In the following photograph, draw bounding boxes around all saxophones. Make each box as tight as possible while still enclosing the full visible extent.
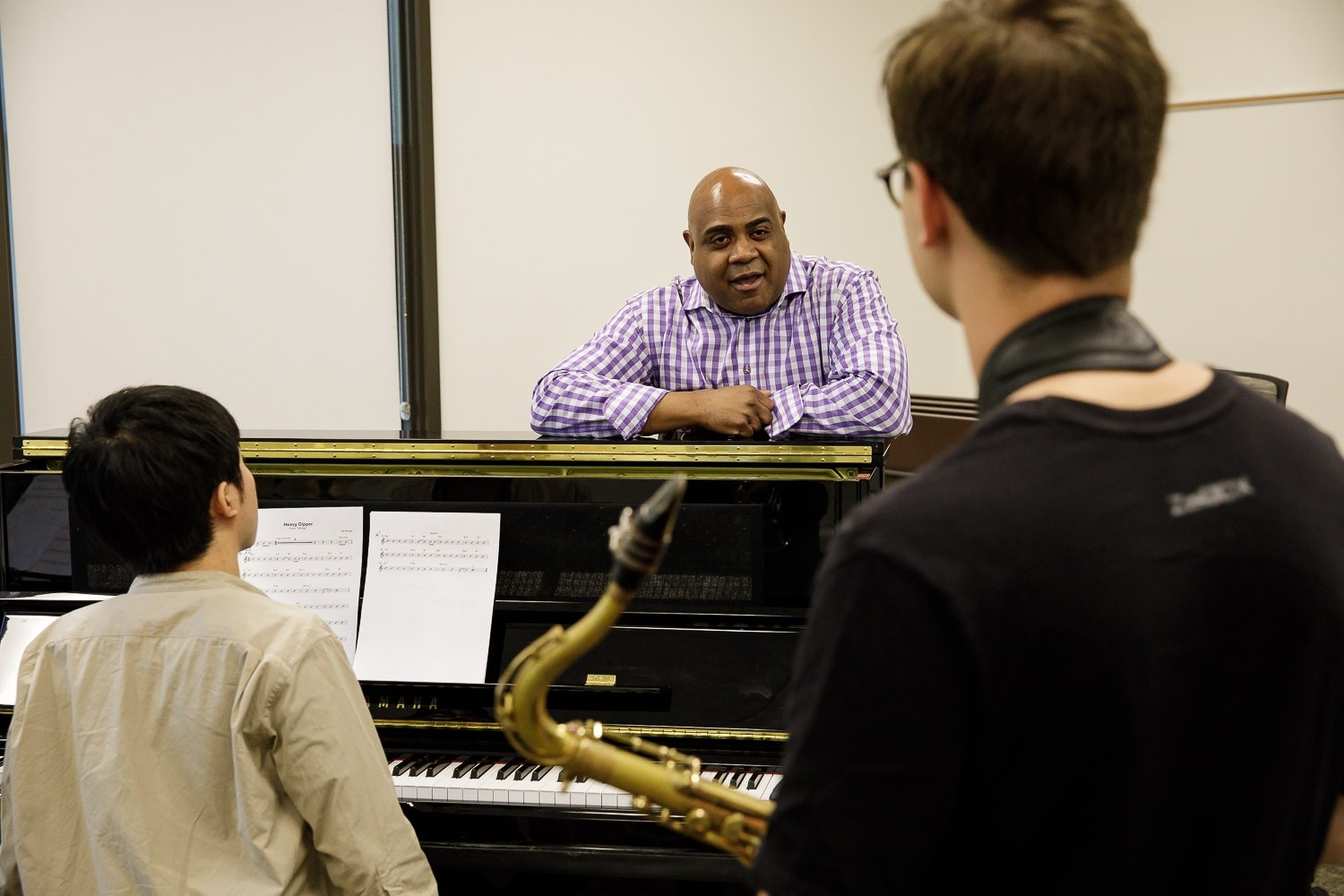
[493,465,770,869]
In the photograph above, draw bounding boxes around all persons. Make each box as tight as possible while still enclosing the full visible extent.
[0,384,438,896]
[750,0,1344,896]
[530,166,913,438]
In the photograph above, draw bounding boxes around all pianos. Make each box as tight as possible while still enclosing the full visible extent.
[54,428,892,894]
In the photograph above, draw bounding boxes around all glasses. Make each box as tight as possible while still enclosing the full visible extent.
[878,155,938,210]
